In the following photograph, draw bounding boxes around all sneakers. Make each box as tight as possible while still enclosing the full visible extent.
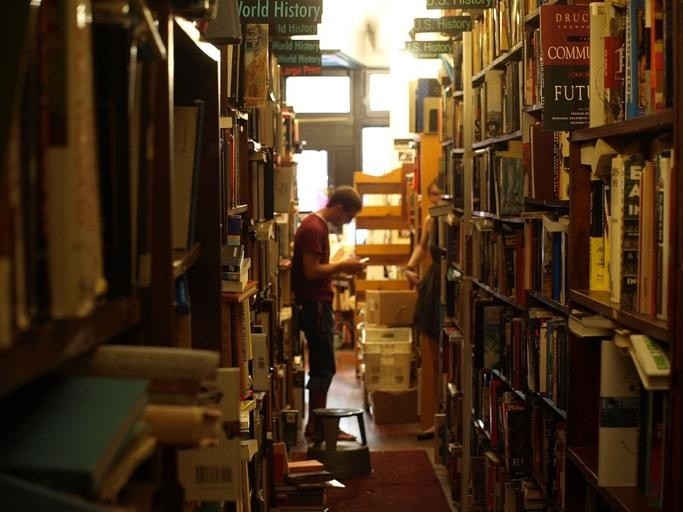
[416,429,436,441]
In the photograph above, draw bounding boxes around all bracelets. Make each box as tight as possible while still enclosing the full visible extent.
[405,264,415,271]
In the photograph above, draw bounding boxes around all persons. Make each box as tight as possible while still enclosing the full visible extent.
[402,172,439,441]
[291,184,368,443]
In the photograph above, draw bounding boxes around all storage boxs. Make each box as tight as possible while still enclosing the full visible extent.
[361,324,415,392]
[360,287,417,324]
[367,390,420,426]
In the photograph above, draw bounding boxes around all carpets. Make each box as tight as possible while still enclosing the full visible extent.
[289,449,451,511]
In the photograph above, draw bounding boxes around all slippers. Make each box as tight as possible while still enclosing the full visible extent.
[305,426,358,442]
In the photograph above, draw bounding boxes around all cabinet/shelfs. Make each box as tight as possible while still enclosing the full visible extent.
[354,132,413,429]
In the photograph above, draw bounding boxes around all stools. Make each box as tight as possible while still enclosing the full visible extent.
[312,405,374,479]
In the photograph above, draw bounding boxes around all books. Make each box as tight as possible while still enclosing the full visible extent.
[0,0,349,511]
[437,0,676,511]
